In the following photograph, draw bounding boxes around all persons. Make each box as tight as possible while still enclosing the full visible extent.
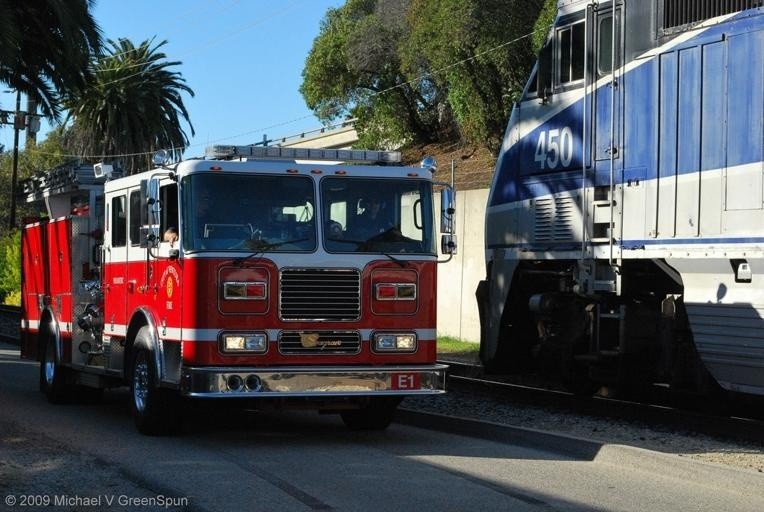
[344,195,393,234]
[162,187,226,249]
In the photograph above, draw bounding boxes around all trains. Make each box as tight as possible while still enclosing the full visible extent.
[474,0,764,406]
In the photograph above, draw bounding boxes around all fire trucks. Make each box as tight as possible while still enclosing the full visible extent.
[17,138,463,438]
